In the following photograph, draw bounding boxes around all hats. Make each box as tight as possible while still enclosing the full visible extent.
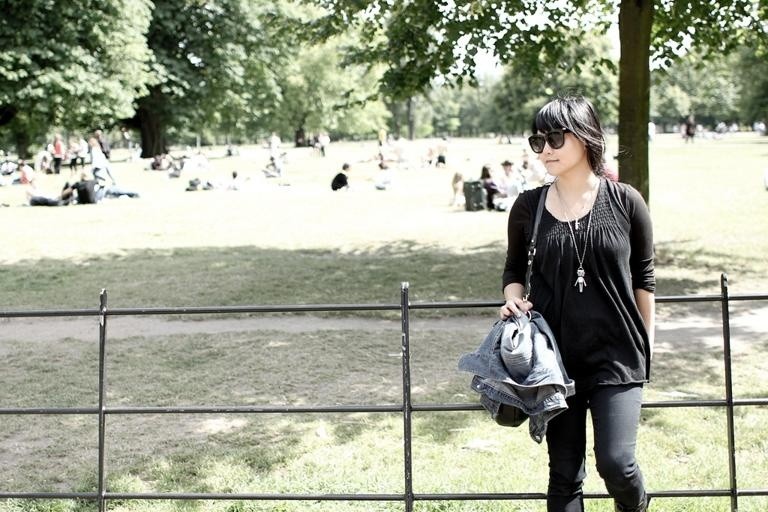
[502,161,511,165]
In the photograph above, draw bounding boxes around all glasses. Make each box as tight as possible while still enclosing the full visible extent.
[528,128,571,152]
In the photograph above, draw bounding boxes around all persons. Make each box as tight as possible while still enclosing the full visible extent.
[646,112,768,144]
[1,127,542,213]
[498,91,658,512]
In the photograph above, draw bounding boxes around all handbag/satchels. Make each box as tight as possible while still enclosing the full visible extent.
[480,394,529,428]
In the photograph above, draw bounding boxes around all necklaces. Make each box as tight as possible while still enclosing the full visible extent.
[554,175,597,295]
